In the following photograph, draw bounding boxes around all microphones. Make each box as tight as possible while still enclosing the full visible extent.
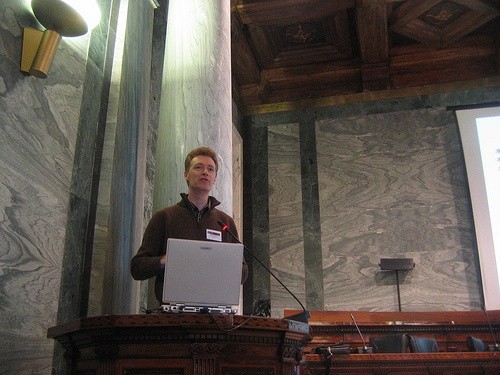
[350,314,373,353]
[484,307,500,351]
[216,219,311,323]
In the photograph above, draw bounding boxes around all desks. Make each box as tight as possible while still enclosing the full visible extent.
[47,313,309,375]
[304,351,500,375]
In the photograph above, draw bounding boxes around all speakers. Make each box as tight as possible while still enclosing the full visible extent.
[380,258,414,270]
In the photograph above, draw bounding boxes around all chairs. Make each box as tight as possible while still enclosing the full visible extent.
[466,335,486,352]
[371,334,409,353]
[410,336,439,352]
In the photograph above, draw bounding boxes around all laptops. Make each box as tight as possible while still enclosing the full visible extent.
[145,238,243,314]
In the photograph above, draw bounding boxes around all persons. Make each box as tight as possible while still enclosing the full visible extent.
[131,147,248,308]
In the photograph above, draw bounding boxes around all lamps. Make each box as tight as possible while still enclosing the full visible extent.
[20,0,102,79]
